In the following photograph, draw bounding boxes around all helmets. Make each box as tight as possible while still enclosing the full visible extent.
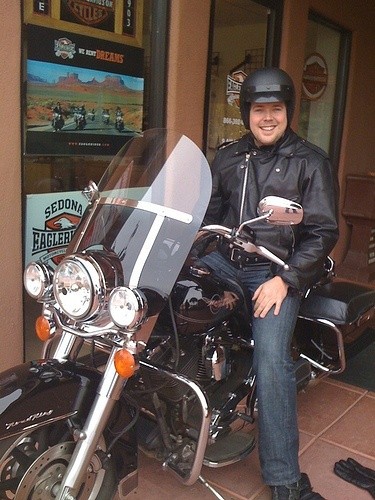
[240,67,295,129]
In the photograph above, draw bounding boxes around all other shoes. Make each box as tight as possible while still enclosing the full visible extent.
[270,473,326,500]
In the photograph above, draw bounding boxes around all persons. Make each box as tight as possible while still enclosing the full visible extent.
[161,66,341,500]
[52,102,123,129]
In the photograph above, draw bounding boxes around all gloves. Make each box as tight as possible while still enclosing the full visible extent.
[334,458,375,499]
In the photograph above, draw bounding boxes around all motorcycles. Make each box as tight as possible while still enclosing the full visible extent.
[73,112,110,130]
[0,128,375,500]
[50,107,66,131]
[114,114,125,132]
